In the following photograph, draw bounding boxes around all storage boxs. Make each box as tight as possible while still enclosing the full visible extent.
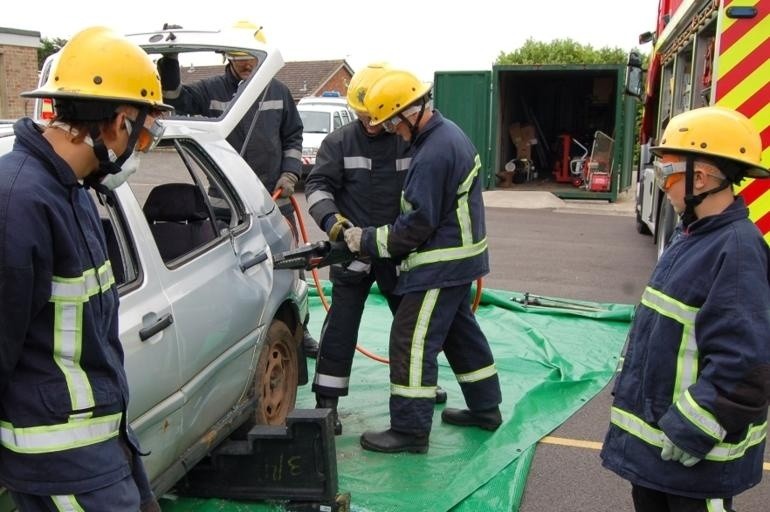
[510,122,537,160]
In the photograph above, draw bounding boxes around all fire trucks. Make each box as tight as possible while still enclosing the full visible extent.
[621,0,769,269]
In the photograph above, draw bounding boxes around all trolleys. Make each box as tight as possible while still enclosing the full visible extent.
[566,135,588,188]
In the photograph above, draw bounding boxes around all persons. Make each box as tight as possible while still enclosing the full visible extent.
[342,69,503,458]
[158,21,320,360]
[304,64,451,438]
[599,107,770,512]
[0,27,177,512]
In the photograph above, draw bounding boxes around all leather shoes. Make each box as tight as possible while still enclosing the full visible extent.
[436,389,447,407]
[441,408,500,432]
[360,430,429,455]
[303,330,320,359]
[318,407,342,436]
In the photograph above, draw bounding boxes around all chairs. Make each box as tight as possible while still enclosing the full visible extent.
[106,183,229,283]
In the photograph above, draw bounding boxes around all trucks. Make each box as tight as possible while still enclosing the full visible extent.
[293,88,360,182]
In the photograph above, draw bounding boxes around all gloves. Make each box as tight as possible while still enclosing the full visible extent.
[276,172,298,198]
[323,214,353,242]
[344,227,362,253]
[156,56,180,91]
[660,432,701,467]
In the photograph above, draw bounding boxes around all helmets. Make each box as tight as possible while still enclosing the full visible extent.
[224,22,267,59]
[346,61,391,115]
[21,25,176,115]
[648,106,769,178]
[362,69,435,126]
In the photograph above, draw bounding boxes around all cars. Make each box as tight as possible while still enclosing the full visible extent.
[1,22,321,511]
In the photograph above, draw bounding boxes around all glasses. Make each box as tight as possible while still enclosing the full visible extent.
[224,55,260,71]
[653,153,726,191]
[382,101,433,134]
[112,104,165,153]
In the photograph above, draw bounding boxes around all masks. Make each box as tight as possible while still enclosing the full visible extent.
[100,151,140,190]
